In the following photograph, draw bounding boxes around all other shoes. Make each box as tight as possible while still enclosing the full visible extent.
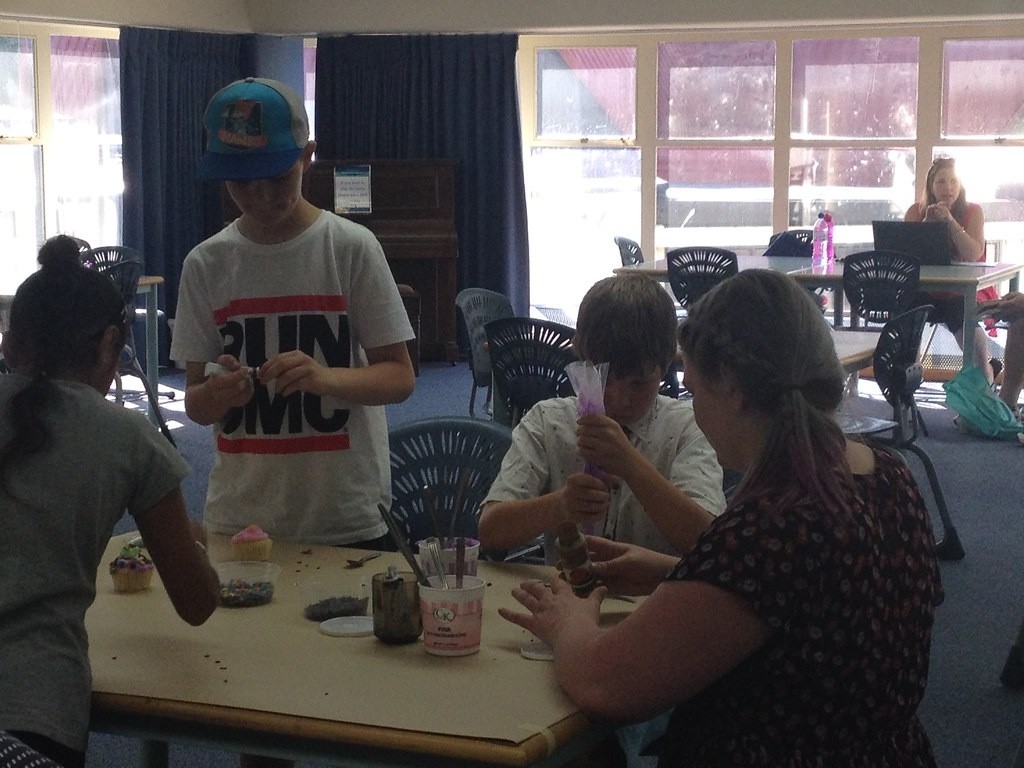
[951,404,1020,434]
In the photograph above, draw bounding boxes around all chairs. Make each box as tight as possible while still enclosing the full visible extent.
[53,228,1003,565]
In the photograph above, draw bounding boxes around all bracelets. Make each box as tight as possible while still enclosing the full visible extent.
[952,227,965,242]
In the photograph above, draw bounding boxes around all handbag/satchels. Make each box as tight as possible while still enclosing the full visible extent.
[942,365,1024,441]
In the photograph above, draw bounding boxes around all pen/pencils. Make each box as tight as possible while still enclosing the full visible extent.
[429,544,450,589]
[377,503,433,588]
[448,469,471,547]
[456,538,466,589]
[422,490,446,550]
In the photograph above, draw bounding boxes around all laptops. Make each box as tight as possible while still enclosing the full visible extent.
[872,220,952,265]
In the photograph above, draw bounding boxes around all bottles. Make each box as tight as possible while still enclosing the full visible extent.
[812,213,828,262]
[555,522,597,594]
[824,214,834,259]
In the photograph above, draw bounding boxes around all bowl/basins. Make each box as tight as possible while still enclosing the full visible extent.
[215,560,282,607]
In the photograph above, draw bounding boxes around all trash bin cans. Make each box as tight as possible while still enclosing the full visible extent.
[655,177,672,226]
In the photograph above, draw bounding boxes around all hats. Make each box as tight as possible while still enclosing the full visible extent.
[200,76,309,181]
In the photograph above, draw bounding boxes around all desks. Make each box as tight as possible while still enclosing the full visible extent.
[483,315,688,399]
[135,274,164,429]
[612,255,845,328]
[789,260,1024,433]
[85,525,665,768]
[830,331,883,393]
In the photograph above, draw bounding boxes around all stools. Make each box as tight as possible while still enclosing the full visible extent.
[396,283,423,378]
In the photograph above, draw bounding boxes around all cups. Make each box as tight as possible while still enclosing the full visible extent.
[419,536,481,577]
[418,575,486,657]
[372,571,423,645]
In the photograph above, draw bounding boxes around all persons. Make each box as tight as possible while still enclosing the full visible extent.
[477,272,726,557]
[0,235,222,767]
[873,157,1024,428]
[497,268,938,768]
[170,76,416,552]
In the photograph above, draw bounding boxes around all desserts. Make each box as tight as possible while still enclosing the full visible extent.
[109,546,154,592]
[230,524,273,561]
